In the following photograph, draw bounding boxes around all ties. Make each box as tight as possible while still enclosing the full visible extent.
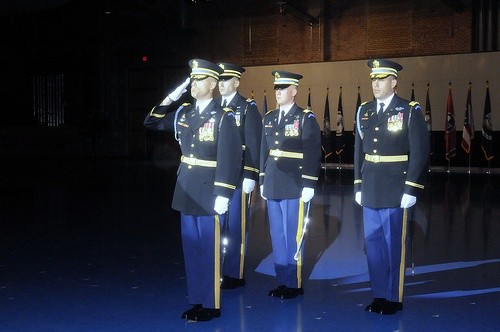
[195,107,199,114]
[280,110,285,119]
[377,103,385,124]
[222,100,227,107]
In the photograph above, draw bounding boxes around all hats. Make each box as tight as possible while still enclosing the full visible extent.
[215,63,245,81]
[367,58,403,79]
[188,59,224,81]
[272,70,304,89]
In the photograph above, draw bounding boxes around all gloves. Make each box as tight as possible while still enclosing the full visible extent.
[259,185,267,201]
[302,186,314,203]
[168,77,190,102]
[400,193,417,208]
[355,191,363,206]
[214,195,229,215]
[243,178,256,194]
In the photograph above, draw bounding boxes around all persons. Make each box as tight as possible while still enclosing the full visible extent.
[144,58,242,322]
[353,57,430,315]
[215,62,263,289]
[259,71,321,300]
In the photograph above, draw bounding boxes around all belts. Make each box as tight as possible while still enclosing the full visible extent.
[269,149,303,159]
[364,154,408,163]
[180,155,217,167]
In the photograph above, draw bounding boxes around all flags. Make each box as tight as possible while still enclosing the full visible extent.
[461,86,475,153]
[250,88,432,154]
[445,87,457,160]
[481,84,493,165]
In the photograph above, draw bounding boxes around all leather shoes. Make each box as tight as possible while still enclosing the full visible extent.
[181,304,201,319]
[191,307,219,322]
[279,287,305,300]
[268,285,286,296]
[364,297,385,312]
[219,275,244,290]
[380,300,403,315]
